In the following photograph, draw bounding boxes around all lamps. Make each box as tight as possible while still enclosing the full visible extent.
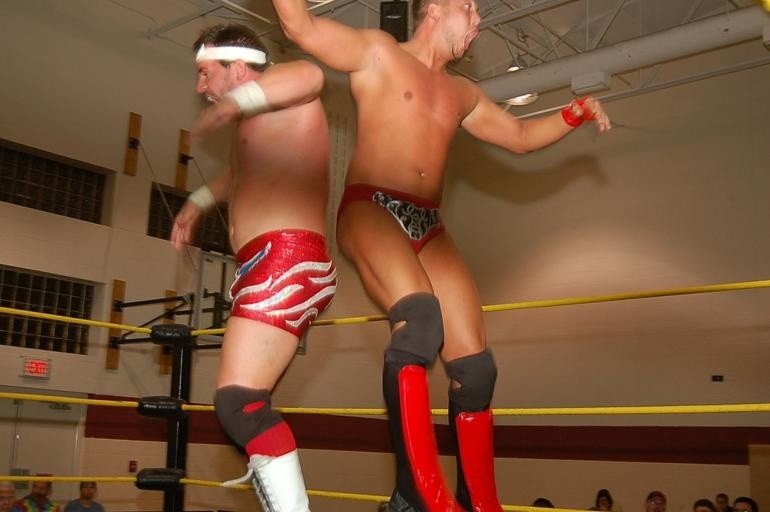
[499,59,538,106]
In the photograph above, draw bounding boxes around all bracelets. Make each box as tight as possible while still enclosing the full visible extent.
[222,79,269,118]
[188,184,217,214]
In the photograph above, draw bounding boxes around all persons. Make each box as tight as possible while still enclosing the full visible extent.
[63,480,105,512]
[693,492,759,511]
[11,474,61,512]
[166,22,340,512]
[588,488,612,511]
[271,1,612,512]
[645,491,667,512]
